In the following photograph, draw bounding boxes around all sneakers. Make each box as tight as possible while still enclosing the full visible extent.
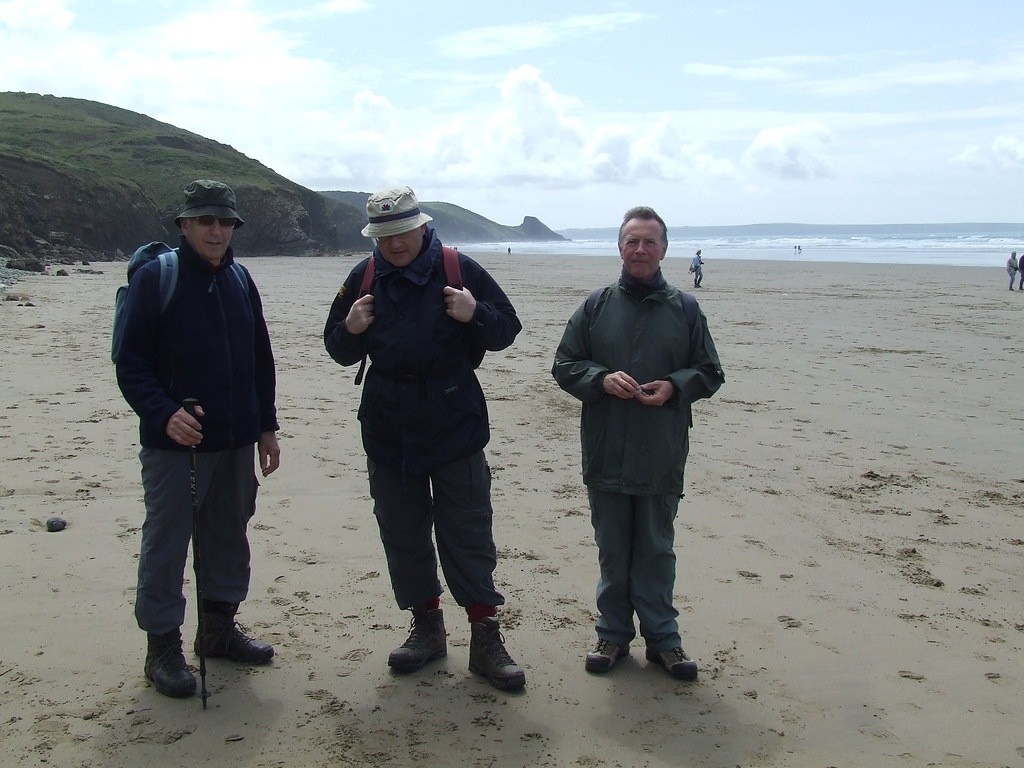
[585,638,629,673]
[646,646,698,681]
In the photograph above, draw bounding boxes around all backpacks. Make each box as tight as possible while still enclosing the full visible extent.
[111,242,249,364]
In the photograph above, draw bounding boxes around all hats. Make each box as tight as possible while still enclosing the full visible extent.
[696,249,701,255]
[174,180,245,230]
[361,186,433,238]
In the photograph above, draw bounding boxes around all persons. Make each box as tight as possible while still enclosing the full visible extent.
[552,206,725,682]
[692,248,704,288]
[1007,251,1024,291]
[111,179,280,699]
[508,247,511,255]
[322,186,526,690]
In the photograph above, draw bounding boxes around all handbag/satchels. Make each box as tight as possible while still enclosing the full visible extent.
[1015,267,1018,271]
[689,267,697,272]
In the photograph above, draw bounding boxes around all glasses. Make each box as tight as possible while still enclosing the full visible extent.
[190,215,237,226]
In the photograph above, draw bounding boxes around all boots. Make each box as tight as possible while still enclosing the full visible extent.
[194,598,274,665]
[694,278,702,288]
[144,629,197,698]
[468,616,526,693]
[388,609,447,673]
